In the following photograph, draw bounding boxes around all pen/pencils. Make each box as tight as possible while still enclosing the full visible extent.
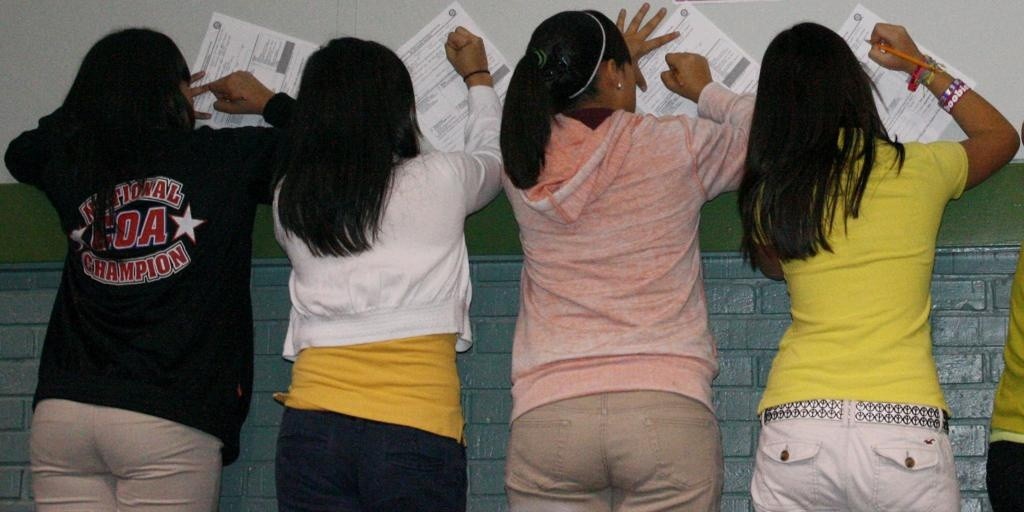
[670,65,685,86]
[223,96,247,101]
[455,41,472,51]
[864,39,935,71]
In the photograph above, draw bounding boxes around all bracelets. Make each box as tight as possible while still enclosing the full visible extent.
[464,70,491,82]
[937,80,970,114]
[908,50,933,91]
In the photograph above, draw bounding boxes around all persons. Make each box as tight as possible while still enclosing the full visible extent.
[736,21,1020,512]
[497,3,755,512]
[985,240,1024,512]
[271,27,504,511]
[4,28,296,512]
